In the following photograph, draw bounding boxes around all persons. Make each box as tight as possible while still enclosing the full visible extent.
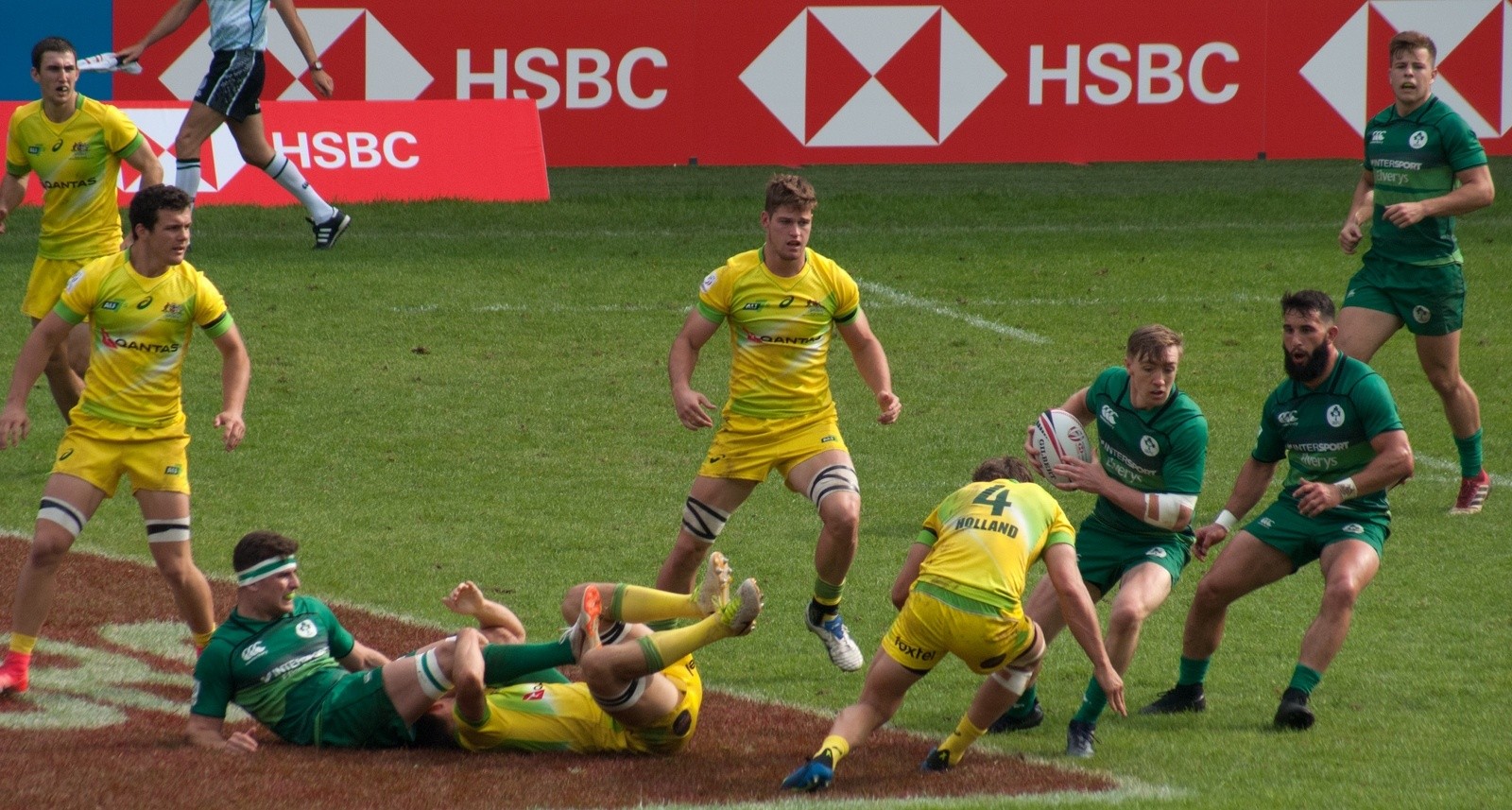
[1137,289,1415,732]
[1333,30,1496,514]
[109,0,352,255]
[779,456,1128,793]
[646,174,902,672]
[0,184,251,695]
[186,530,765,759]
[0,36,164,426]
[985,324,1210,759]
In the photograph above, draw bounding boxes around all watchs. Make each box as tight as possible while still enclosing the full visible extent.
[309,60,323,71]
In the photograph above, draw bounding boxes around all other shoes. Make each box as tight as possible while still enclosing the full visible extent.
[0,660,27,696]
[197,647,203,657]
[558,584,602,663]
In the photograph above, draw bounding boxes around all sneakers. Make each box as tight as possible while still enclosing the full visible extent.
[781,749,834,793]
[1449,470,1491,515]
[1065,720,1103,759]
[921,745,951,773]
[803,598,864,673]
[709,578,763,637]
[1137,682,1206,715]
[988,697,1044,734]
[1274,687,1315,731]
[304,207,349,250]
[686,551,732,617]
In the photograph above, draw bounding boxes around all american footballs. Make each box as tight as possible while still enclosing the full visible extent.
[1031,410,1093,490]
